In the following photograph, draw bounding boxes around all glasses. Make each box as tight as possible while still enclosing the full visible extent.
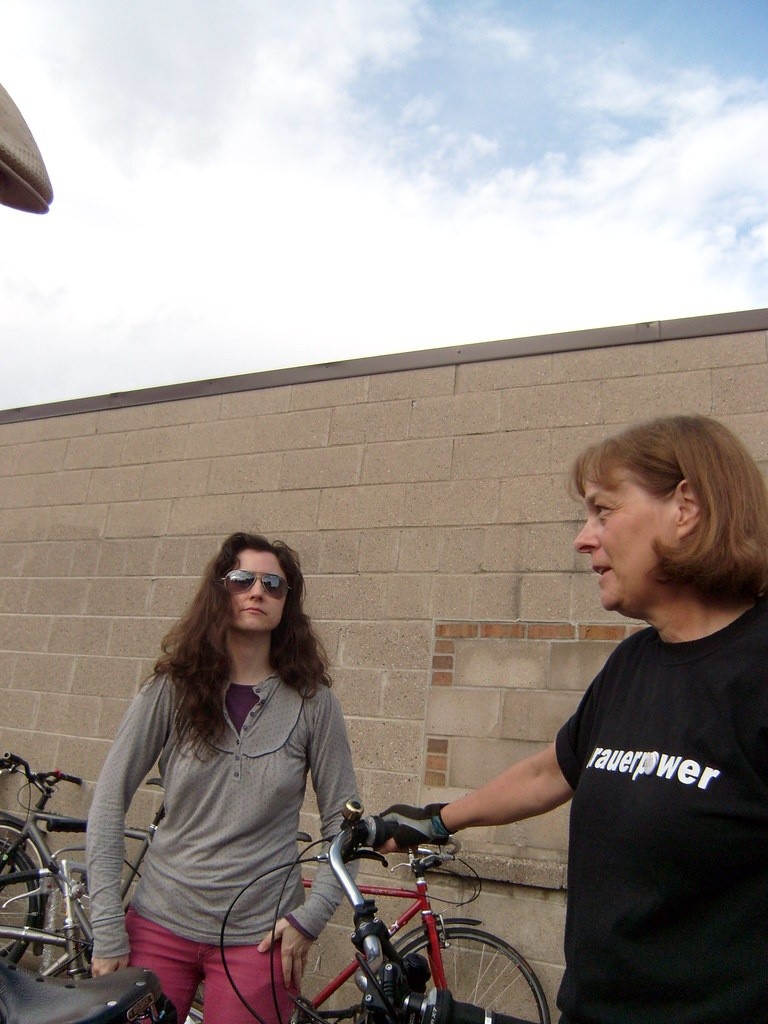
[220,569,292,600]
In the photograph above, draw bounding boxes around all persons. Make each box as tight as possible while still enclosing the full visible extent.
[377,416,768,1024]
[86,530,363,1024]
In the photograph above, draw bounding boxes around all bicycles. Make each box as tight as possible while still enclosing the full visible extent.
[0,748,553,1024]
